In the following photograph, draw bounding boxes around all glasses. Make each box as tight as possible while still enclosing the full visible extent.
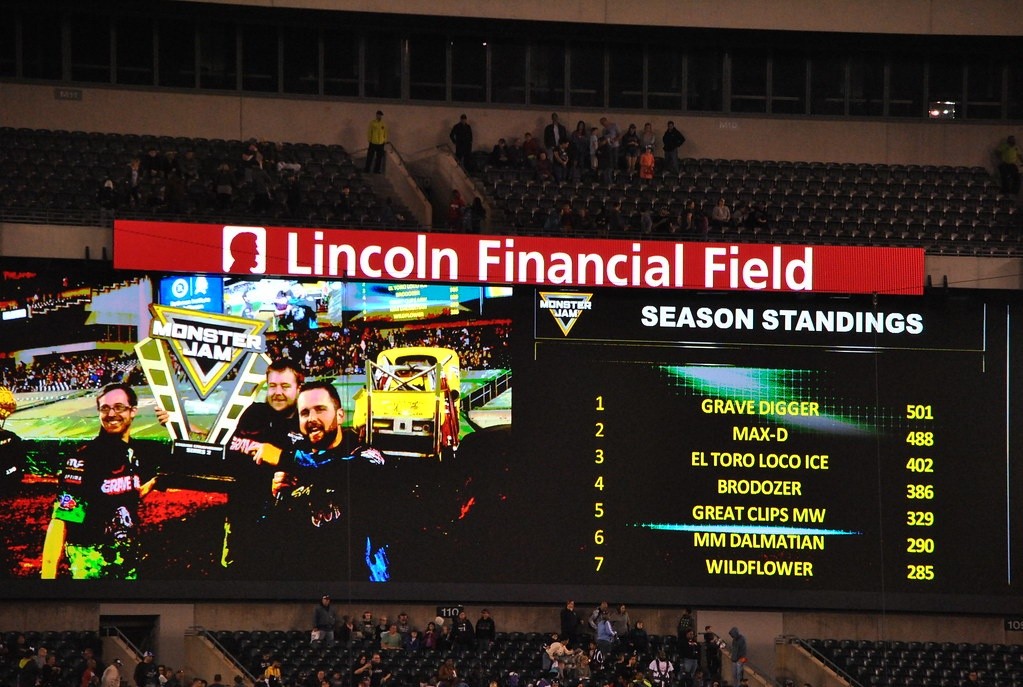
[98,403,132,413]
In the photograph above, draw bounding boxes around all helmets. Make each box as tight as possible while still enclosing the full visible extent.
[112,659,123,666]
[63,654,88,672]
[143,650,155,657]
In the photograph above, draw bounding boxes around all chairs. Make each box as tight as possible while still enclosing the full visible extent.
[0,123,421,232]
[803,639,1023,687]
[464,150,1023,257]
[0,630,105,687]
[202,628,682,687]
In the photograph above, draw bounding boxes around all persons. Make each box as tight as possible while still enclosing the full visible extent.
[448,190,486,232]
[711,198,730,222]
[545,200,710,240]
[662,121,685,160]
[509,139,523,169]
[544,113,656,184]
[535,152,554,183]
[450,114,473,160]
[0,593,747,687]
[0,268,509,560]
[997,135,1023,197]
[522,133,541,157]
[341,186,356,208]
[125,140,275,207]
[364,111,387,175]
[494,138,508,168]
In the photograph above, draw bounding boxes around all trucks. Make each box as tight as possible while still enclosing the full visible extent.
[352,347,463,454]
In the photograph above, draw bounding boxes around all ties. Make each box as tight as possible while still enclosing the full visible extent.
[128,447,134,463]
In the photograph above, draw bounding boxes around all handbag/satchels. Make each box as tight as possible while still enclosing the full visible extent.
[309,628,320,643]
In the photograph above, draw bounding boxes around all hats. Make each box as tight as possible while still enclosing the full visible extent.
[399,610,408,617]
